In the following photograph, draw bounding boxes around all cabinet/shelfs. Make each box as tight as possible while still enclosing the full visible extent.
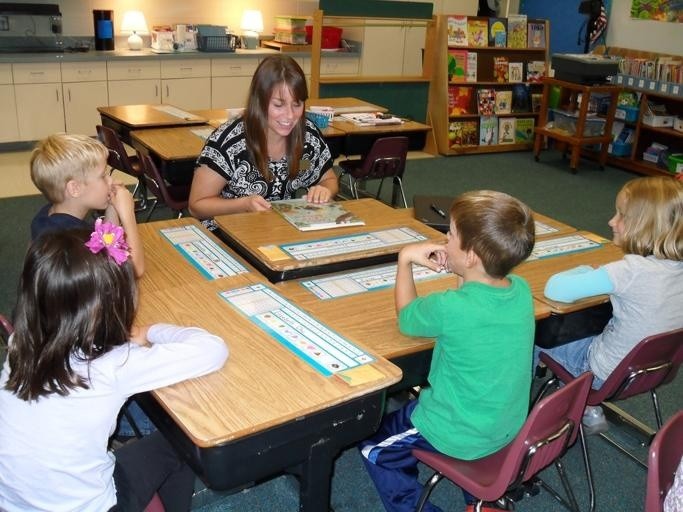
[104,60,211,113]
[423,15,552,157]
[0,63,18,149]
[260,57,358,95]
[324,13,430,79]
[10,60,107,143]
[207,58,259,111]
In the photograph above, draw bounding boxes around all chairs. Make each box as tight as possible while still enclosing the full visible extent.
[0,316,142,445]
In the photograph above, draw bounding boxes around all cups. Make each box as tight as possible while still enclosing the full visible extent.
[93,10,115,51]
[495,31,506,48]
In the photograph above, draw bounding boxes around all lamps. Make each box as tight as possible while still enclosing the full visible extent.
[236,9,266,49]
[118,10,148,50]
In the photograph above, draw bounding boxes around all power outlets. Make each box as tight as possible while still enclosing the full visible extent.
[48,16,64,35]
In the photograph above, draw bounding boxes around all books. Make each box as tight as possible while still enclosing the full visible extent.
[447,13,547,147]
[611,54,682,116]
[267,199,365,232]
[341,112,403,128]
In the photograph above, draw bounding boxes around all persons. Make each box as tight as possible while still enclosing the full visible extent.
[28,131,146,279]
[186,53,339,234]
[357,188,536,512]
[532,174,682,436]
[0,231,229,512]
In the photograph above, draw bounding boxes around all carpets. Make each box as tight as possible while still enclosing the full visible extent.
[0,150,683,510]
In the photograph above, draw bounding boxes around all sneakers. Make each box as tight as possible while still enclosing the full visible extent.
[576,401,613,441]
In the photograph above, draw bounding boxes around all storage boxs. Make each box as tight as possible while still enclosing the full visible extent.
[304,23,344,49]
[272,26,306,44]
[550,52,619,85]
[270,16,307,28]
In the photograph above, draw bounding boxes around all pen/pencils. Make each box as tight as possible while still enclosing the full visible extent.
[353,117,362,121]
[431,204,447,218]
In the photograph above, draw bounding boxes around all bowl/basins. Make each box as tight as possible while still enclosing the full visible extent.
[305,25,342,50]
